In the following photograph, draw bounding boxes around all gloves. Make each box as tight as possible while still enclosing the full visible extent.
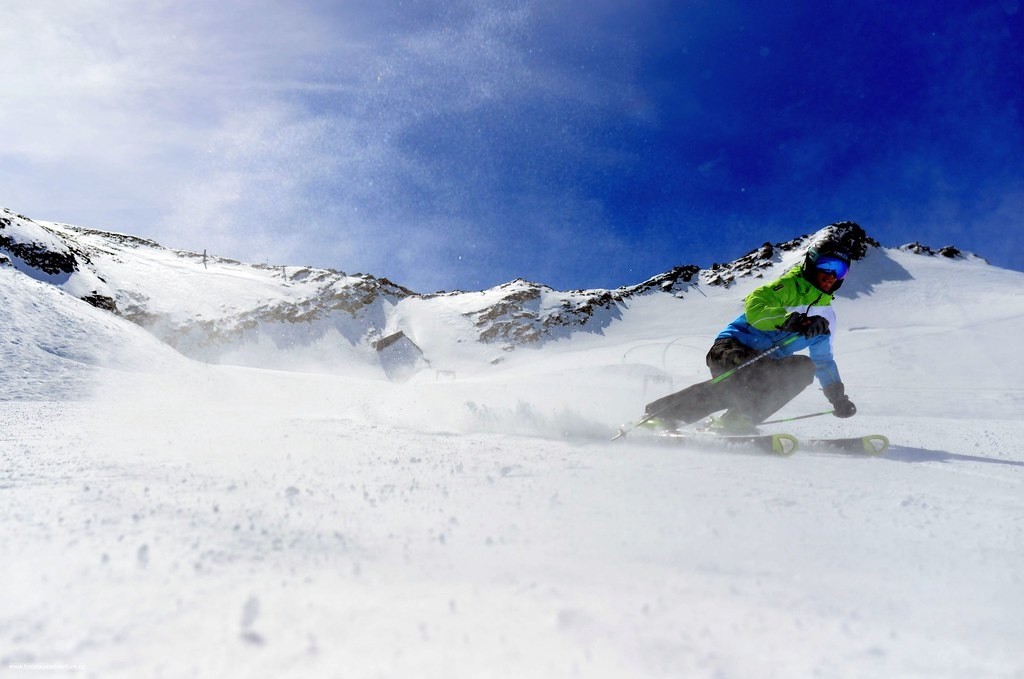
[776,311,830,341]
[822,383,857,418]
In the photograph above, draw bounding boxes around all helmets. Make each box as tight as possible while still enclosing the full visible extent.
[805,239,851,295]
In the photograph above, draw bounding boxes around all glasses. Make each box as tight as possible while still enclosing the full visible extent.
[814,253,850,281]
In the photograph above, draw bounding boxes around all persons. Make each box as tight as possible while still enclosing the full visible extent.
[639,240,857,436]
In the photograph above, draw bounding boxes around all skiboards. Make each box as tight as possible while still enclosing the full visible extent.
[617,434,887,455]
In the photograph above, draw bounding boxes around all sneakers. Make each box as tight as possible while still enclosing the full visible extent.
[715,413,760,435]
[635,413,676,433]
